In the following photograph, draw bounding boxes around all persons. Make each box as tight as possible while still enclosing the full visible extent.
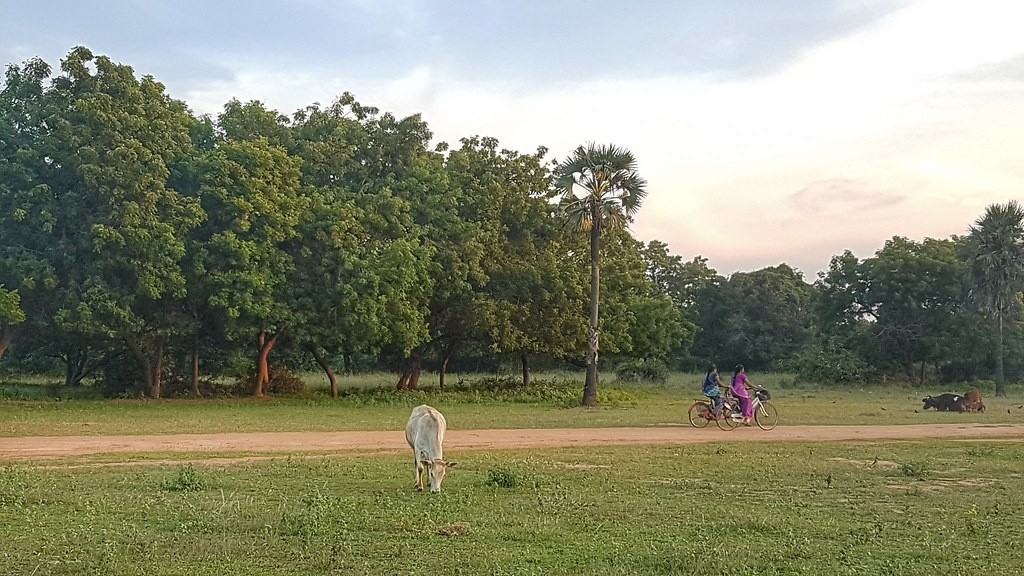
[702,364,732,419]
[731,364,764,427]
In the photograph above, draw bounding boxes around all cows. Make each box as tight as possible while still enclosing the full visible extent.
[405,404,459,494]
[922,390,986,414]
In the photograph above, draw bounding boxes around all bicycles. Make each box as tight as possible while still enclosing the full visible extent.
[714,386,779,430]
[687,387,746,428]
